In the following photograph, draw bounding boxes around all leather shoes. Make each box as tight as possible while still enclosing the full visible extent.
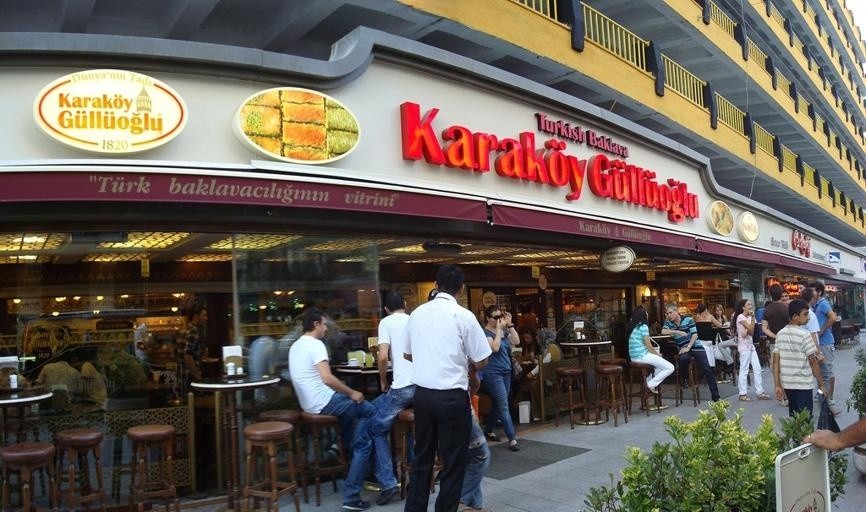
[509,440,520,451]
[484,431,499,442]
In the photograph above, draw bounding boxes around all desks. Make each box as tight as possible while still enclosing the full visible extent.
[1,386,56,440]
[559,341,613,427]
[333,360,394,398]
[187,372,284,511]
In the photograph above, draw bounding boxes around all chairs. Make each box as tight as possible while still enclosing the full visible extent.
[0,341,618,408]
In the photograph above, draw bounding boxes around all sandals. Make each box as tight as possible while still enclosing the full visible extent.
[739,393,771,401]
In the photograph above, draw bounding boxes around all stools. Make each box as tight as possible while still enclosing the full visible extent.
[555,364,591,431]
[49,426,105,510]
[392,405,444,499]
[629,359,661,417]
[242,419,300,509]
[603,358,629,412]
[259,409,309,503]
[598,364,629,428]
[125,422,181,512]
[1,440,55,512]
[674,352,701,409]
[729,345,752,387]
[299,409,348,508]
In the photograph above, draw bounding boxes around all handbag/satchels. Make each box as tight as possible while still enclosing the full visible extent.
[511,355,523,375]
[518,401,531,424]
[713,332,731,361]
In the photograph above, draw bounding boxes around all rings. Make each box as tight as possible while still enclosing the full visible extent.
[808,435,811,439]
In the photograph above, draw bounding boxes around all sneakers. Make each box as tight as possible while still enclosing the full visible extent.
[648,386,658,394]
[726,362,737,373]
[329,443,342,462]
[377,486,399,504]
[458,503,481,512]
[343,500,370,510]
[827,398,834,406]
[814,392,824,402]
[681,377,688,388]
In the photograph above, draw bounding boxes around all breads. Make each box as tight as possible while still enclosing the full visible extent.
[241,89,358,160]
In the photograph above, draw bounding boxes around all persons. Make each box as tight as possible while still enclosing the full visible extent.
[804,418,866,452]
[288,265,563,510]
[630,303,739,410]
[730,281,837,423]
[2,303,208,410]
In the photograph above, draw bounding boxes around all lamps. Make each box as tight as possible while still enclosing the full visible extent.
[643,287,650,301]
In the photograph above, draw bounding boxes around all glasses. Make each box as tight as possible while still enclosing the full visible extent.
[491,315,503,320]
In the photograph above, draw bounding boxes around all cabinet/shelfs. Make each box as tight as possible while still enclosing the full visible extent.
[660,288,704,322]
